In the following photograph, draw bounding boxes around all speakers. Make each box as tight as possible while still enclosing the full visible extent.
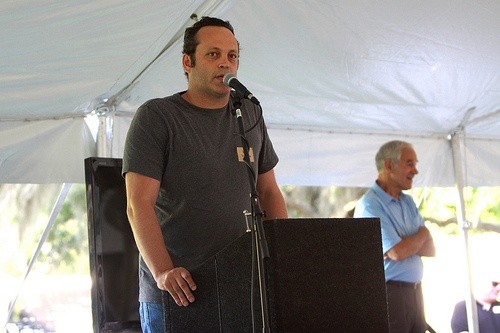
[85,157,142,333]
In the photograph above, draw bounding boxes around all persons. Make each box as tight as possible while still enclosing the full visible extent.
[451,281,500,333]
[353,139,435,333]
[121,15,288,332]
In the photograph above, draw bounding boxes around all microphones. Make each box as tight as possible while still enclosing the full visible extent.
[223,73,260,105]
